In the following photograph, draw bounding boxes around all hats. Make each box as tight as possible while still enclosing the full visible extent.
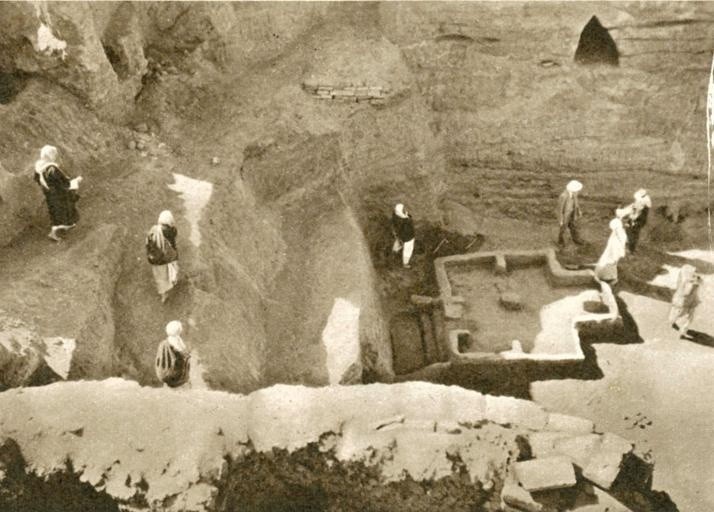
[566,179,583,192]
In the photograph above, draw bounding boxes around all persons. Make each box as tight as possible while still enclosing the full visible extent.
[609,188,655,257]
[143,208,182,304]
[157,316,190,389]
[31,143,86,242]
[664,260,707,342]
[594,218,630,291]
[556,179,590,251]
[388,198,417,272]
